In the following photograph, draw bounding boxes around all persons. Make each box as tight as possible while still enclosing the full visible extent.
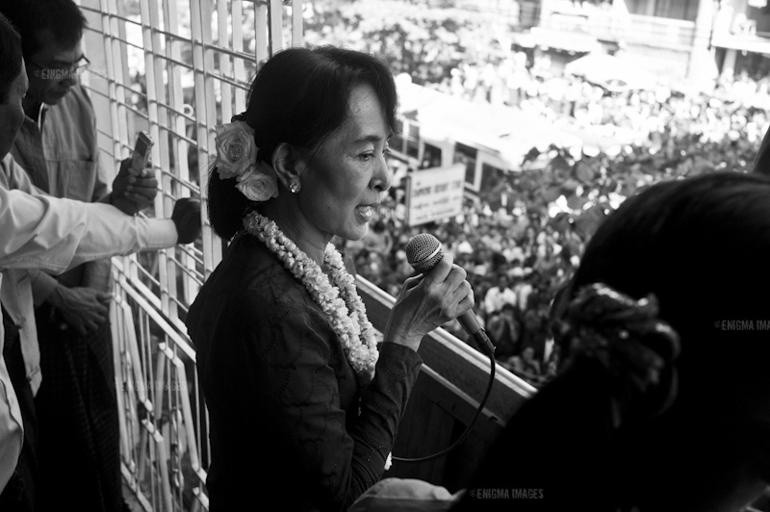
[242,0,770,391]
[348,172,770,512]
[184,47,476,512]
[0,0,202,512]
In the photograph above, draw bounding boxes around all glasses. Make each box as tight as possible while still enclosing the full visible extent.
[30,55,91,79]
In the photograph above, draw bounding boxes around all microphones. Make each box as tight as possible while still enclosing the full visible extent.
[405,232,498,355]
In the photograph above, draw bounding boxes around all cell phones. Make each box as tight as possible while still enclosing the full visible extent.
[126,130,155,177]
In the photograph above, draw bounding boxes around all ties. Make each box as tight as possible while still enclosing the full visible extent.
[0,304,42,472]
